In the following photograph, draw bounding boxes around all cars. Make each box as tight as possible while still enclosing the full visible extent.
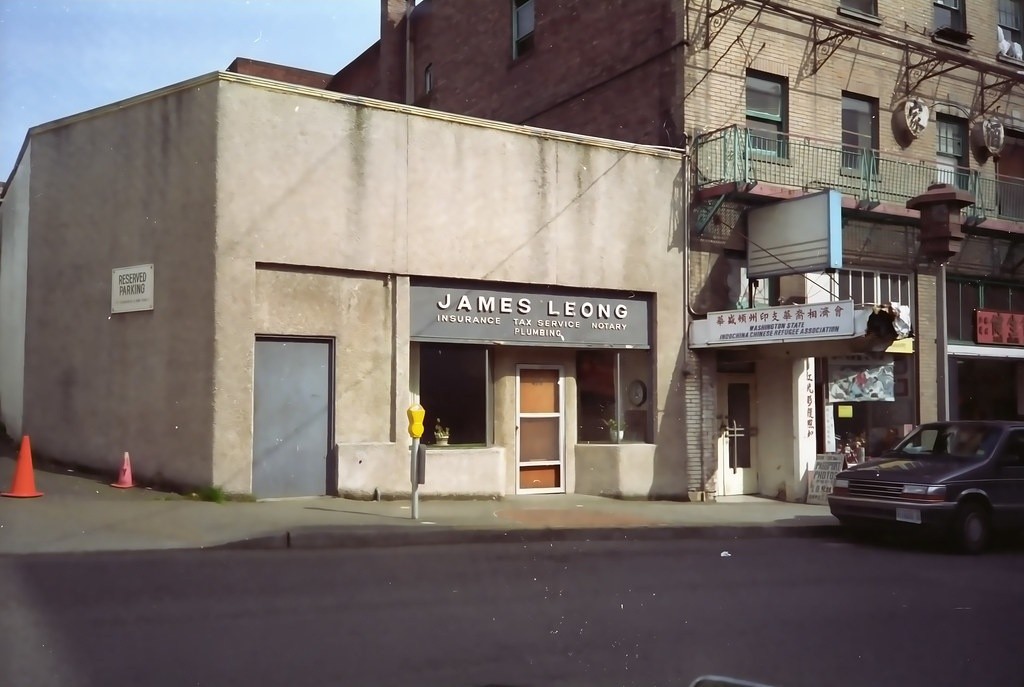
[828,420,1024,553]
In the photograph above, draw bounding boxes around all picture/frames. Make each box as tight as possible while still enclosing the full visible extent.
[894,379,909,397]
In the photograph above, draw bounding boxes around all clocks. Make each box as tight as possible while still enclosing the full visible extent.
[627,380,647,407]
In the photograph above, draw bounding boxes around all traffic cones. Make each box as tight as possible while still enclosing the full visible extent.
[1,436,44,497]
[110,451,136,488]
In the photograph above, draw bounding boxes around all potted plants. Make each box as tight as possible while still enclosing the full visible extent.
[434,418,450,444]
[608,419,628,442]
